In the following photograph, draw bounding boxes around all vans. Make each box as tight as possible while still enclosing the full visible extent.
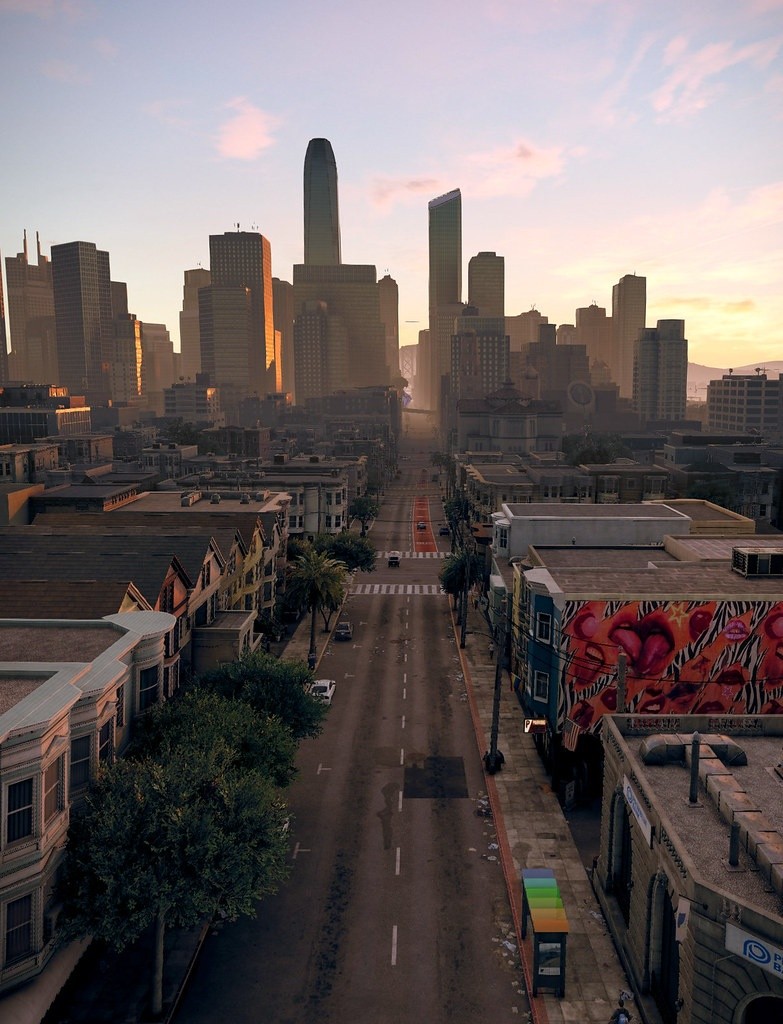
[387,550,400,568]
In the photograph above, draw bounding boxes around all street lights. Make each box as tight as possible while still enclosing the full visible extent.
[462,630,504,775]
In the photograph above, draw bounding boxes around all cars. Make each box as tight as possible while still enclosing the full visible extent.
[334,620,354,642]
[307,678,337,708]
[439,527,449,536]
[417,522,426,530]
